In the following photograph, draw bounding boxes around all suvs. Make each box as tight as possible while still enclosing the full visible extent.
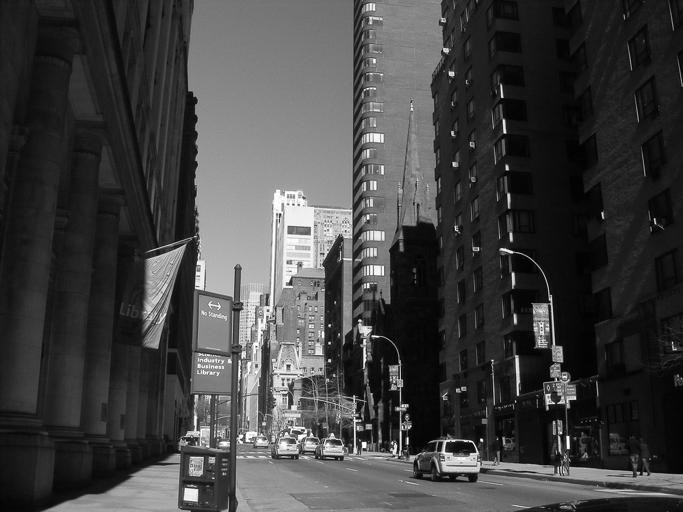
[413,439,481,484]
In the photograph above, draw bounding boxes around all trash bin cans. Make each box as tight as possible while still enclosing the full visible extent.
[178,447,231,512]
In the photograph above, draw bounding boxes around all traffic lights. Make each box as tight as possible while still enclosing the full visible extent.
[297,400,301,408]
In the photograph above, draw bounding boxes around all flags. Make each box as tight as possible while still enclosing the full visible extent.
[114,243,188,351]
[388,365,398,390]
[531,303,551,347]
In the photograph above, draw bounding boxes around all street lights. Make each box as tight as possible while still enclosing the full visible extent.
[496,246,559,384]
[370,333,403,460]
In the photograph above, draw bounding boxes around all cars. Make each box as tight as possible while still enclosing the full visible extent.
[216,425,346,462]
[177,436,199,453]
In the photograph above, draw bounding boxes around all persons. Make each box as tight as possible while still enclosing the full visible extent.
[176,433,196,452]
[551,445,571,475]
[341,434,503,466]
[638,437,652,475]
[625,435,640,477]
